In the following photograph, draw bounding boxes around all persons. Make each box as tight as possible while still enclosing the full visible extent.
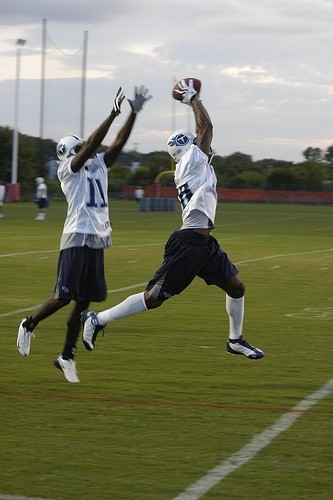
[15,81,153,382]
[0,180,6,219]
[34,177,50,220]
[81,78,269,361]
[134,185,144,205]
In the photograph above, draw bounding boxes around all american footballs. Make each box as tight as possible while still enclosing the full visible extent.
[171,77,201,100]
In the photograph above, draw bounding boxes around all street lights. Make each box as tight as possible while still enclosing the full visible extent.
[11,38,26,185]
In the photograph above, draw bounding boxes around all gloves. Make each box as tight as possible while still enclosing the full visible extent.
[174,80,198,105]
[128,85,152,113]
[112,87,125,116]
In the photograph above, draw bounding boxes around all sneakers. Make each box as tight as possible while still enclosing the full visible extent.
[17,317,33,357]
[54,353,80,383]
[226,335,264,359]
[81,310,105,350]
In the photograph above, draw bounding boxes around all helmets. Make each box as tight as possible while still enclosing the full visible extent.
[56,135,87,161]
[35,177,45,184]
[167,129,199,162]
